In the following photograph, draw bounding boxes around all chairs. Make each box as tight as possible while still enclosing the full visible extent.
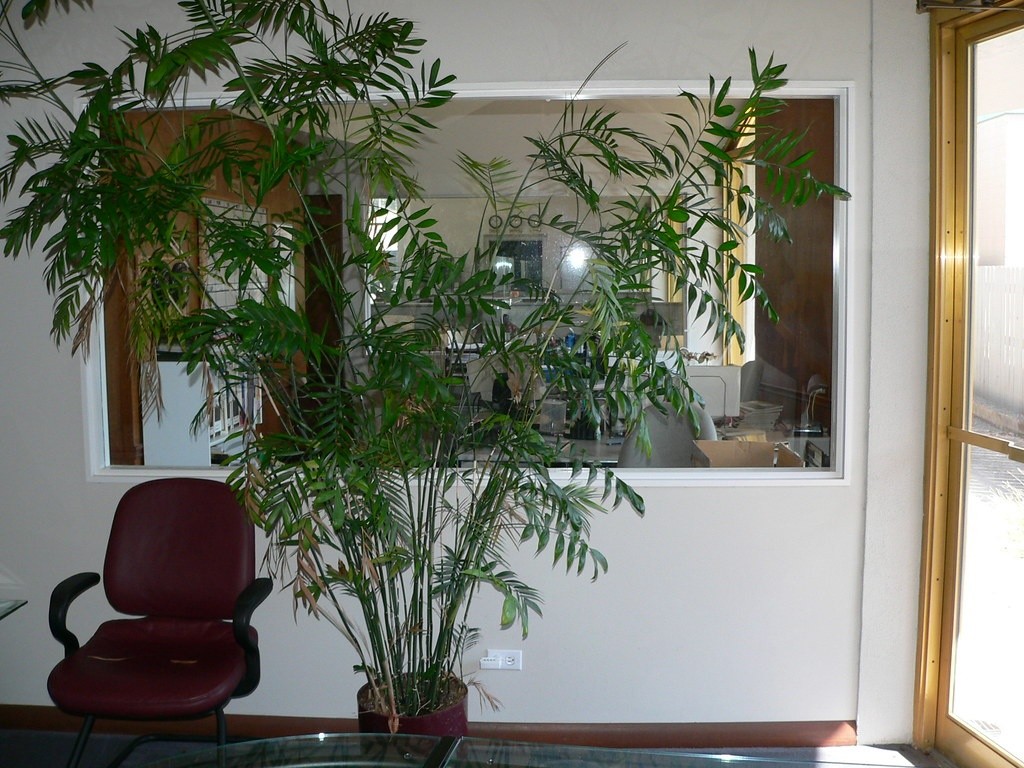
[48,477,274,768]
[618,400,717,469]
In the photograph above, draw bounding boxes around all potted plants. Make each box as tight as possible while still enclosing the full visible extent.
[0,0,851,755]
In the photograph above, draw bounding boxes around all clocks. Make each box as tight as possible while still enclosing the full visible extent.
[509,215,522,228]
[489,215,502,228]
[528,214,542,228]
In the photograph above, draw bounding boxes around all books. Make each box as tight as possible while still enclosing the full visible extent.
[733,400,784,431]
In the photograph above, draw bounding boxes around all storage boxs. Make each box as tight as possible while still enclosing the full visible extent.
[691,438,808,469]
[541,400,566,434]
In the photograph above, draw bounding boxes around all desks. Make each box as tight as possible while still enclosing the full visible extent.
[144,732,910,768]
[457,429,624,468]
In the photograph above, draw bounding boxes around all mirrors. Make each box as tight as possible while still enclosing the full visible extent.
[363,193,690,354]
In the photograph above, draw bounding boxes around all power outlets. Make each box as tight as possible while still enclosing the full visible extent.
[479,649,522,669]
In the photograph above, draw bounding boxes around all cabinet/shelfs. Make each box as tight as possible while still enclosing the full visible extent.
[614,363,742,425]
[140,355,263,468]
